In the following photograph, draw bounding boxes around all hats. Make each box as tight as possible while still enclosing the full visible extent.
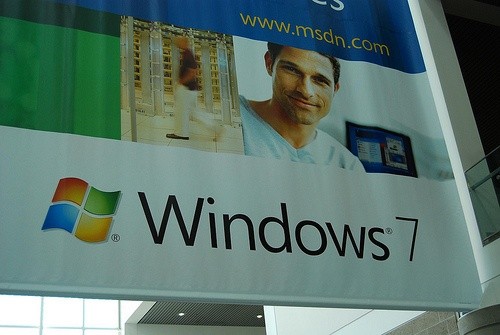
[175,37,188,50]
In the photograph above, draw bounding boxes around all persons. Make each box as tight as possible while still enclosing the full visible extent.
[239,42,366,173]
[165,37,224,141]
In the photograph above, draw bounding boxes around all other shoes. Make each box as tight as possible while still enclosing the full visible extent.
[166,134,189,139]
